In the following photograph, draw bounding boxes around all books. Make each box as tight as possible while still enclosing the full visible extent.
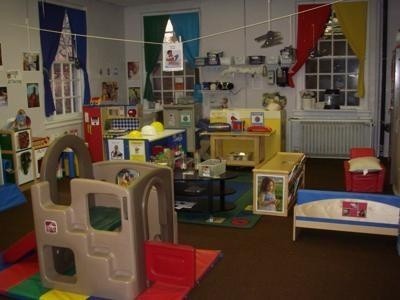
[102,128,130,140]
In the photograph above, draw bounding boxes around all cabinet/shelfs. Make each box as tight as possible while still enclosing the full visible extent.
[82,102,143,162]
[192,63,294,94]
[1,128,51,194]
[163,102,200,153]
[251,151,306,218]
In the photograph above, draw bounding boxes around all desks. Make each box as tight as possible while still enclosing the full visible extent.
[109,128,187,172]
[199,107,286,168]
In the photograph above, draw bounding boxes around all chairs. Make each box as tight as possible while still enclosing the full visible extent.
[343,148,385,194]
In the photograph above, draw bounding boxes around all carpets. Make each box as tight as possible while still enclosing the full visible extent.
[176,170,263,229]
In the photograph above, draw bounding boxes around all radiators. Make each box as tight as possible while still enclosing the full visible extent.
[300,118,374,158]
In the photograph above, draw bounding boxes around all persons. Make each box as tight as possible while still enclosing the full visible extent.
[257,177,281,212]
[28,86,38,107]
[165,49,175,61]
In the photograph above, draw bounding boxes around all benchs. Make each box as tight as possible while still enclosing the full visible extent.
[291,188,400,243]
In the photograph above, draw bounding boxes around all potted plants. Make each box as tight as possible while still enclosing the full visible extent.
[260,92,287,110]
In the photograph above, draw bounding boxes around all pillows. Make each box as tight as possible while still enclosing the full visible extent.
[347,156,382,174]
